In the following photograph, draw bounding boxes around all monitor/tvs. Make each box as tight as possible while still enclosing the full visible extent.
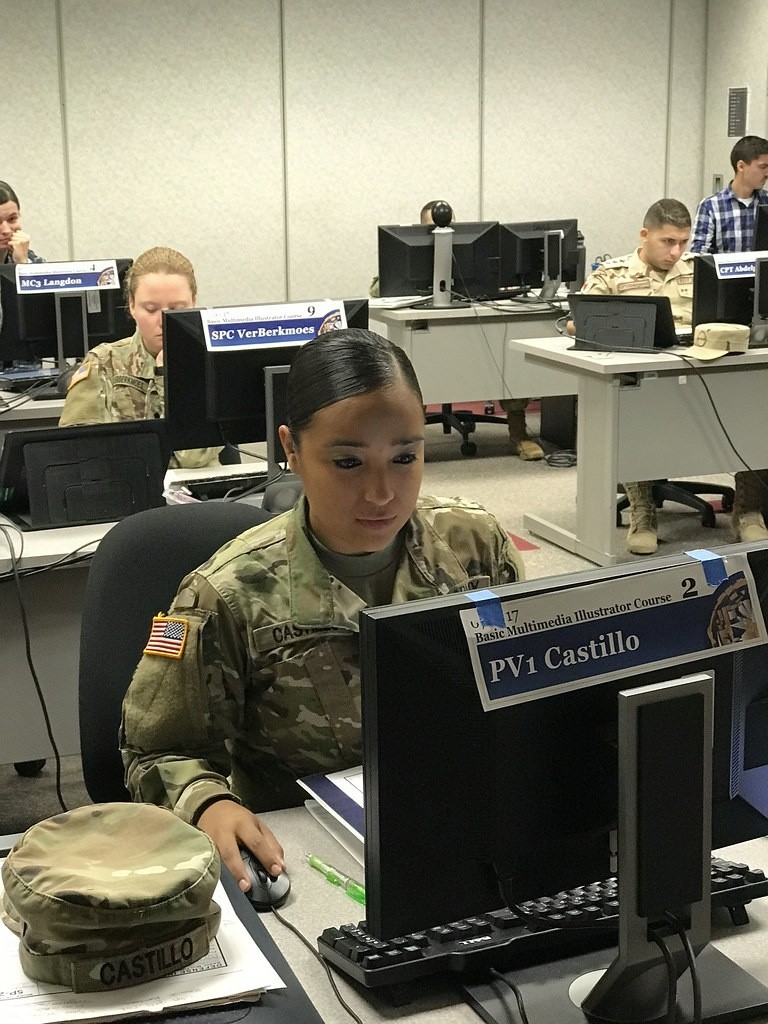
[161,298,375,514]
[0,258,134,400]
[692,250,768,349]
[357,536,768,1024]
[378,219,580,312]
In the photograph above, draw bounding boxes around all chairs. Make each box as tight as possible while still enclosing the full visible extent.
[420,397,736,528]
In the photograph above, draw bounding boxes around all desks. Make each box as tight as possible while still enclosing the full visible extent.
[366,294,579,406]
[243,800,768,1024]
[504,333,768,568]
[0,516,119,775]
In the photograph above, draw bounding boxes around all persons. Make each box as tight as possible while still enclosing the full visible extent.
[57,247,225,469]
[567,198,768,554]
[117,327,528,894]
[368,201,545,461]
[0,180,46,264]
[689,135,768,253]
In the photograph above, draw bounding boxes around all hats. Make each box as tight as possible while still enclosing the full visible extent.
[1,799,222,992]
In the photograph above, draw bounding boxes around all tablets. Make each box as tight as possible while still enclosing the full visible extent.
[568,293,677,348]
[0,418,174,515]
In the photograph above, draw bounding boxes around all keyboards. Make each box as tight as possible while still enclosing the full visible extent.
[461,288,531,303]
[676,333,693,346]
[316,854,768,1009]
[0,366,61,391]
[168,468,291,502]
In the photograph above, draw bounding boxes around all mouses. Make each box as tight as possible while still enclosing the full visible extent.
[239,846,291,911]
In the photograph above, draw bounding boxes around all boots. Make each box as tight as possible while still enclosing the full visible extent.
[507,409,544,460]
[623,481,658,553]
[730,471,768,544]
